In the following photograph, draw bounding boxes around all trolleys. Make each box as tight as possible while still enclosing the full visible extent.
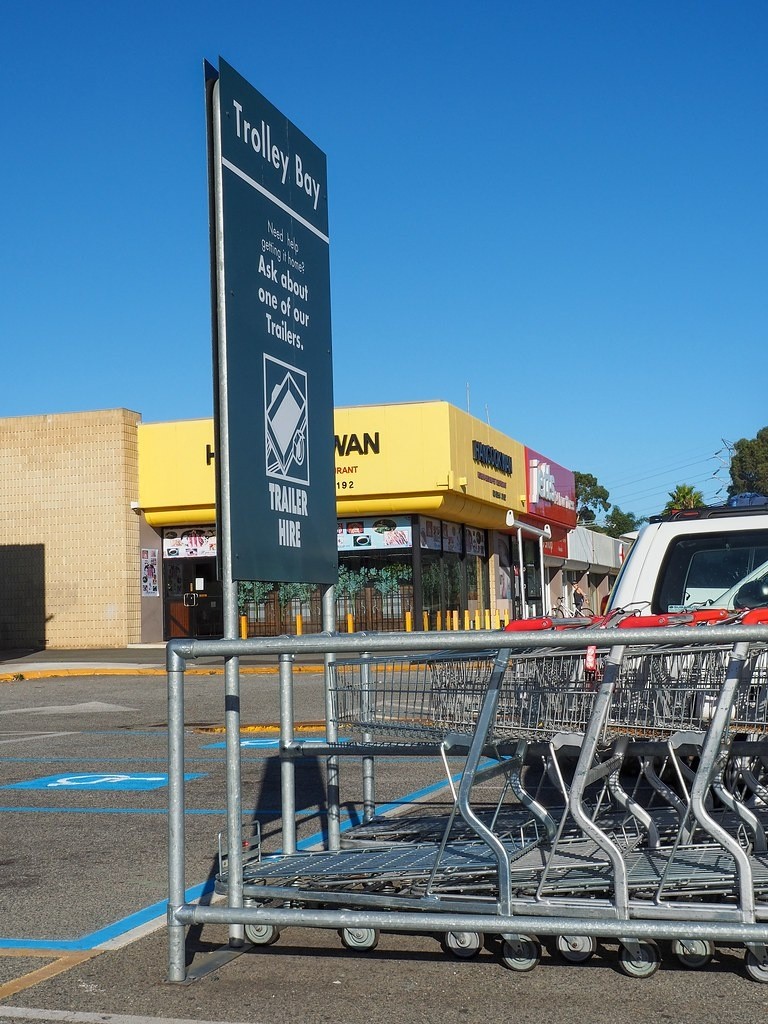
[214,602,768,982]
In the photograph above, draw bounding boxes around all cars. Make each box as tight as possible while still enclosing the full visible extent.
[589,561,768,724]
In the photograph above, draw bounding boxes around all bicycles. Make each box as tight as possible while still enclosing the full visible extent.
[546,596,594,619]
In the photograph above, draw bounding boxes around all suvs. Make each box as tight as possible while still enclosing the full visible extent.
[603,498,768,724]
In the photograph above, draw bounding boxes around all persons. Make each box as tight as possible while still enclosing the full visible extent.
[572,580,588,617]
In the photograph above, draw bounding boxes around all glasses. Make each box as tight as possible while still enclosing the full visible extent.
[572,583,577,585]
